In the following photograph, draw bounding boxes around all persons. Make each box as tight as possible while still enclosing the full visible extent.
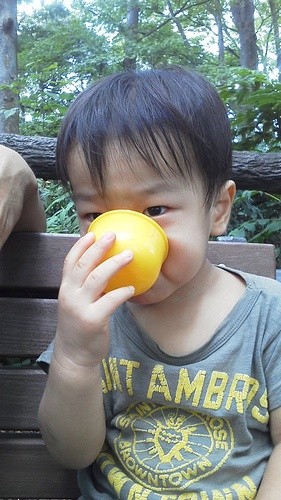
[35,66,281,499]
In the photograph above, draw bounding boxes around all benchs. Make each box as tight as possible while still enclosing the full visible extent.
[0,233,276,500]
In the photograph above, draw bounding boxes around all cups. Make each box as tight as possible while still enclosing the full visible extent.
[87,209,169,297]
